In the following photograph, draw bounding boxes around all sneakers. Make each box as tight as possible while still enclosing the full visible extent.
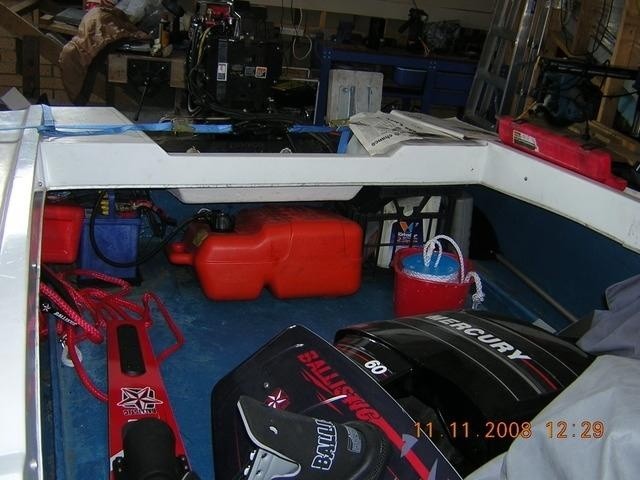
[234,398,389,480]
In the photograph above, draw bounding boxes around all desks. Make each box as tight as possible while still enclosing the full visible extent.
[311,36,482,127]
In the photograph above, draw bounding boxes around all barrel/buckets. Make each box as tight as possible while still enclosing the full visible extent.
[164,204,364,301]
[393,244,476,320]
[39,198,84,265]
